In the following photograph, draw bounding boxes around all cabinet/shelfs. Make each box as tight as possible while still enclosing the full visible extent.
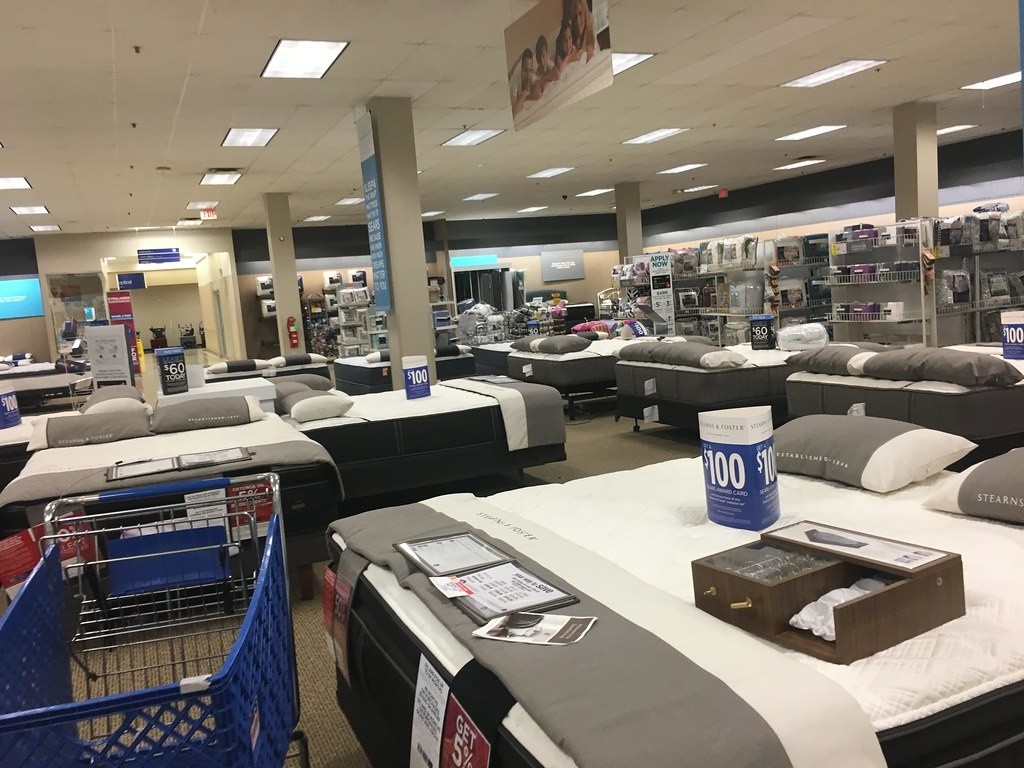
[612,224,1024,347]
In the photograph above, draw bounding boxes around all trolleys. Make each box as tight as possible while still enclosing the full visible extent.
[0,473,311,768]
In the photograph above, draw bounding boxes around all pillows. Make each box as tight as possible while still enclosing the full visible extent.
[0,330,747,450]
[923,446,1024,523]
[783,344,877,374]
[864,348,1024,388]
[770,413,978,492]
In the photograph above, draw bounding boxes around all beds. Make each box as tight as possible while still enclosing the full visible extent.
[0,336,1024,768]
